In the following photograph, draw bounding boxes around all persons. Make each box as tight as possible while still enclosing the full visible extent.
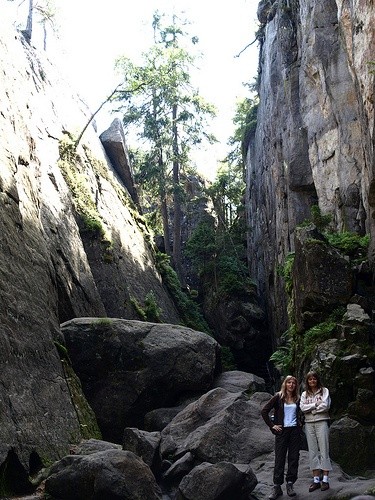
[296,369,333,493]
[261,373,303,499]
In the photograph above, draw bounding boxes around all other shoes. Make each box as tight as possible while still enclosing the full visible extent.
[309,482,321,492]
[321,481,329,491]
[286,483,296,497]
[269,485,283,500]
